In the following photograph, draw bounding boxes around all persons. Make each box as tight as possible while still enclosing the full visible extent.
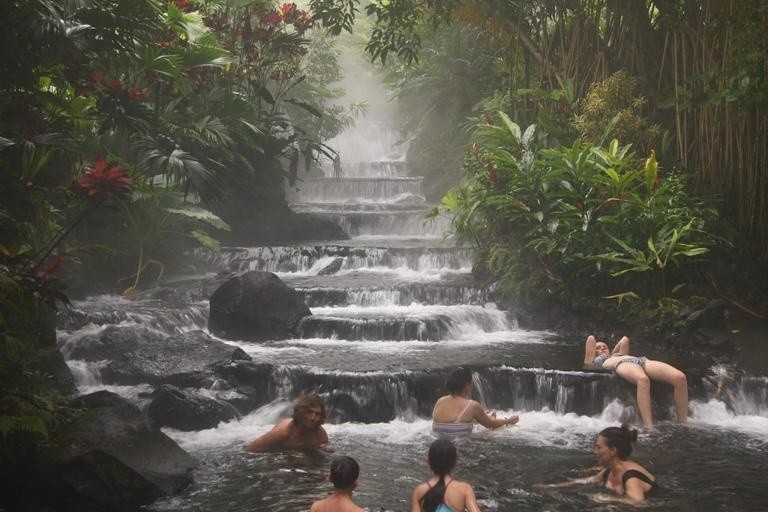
[411,438,481,512]
[583,334,688,430]
[308,456,367,512]
[432,366,520,434]
[531,423,669,505]
[243,395,328,454]
